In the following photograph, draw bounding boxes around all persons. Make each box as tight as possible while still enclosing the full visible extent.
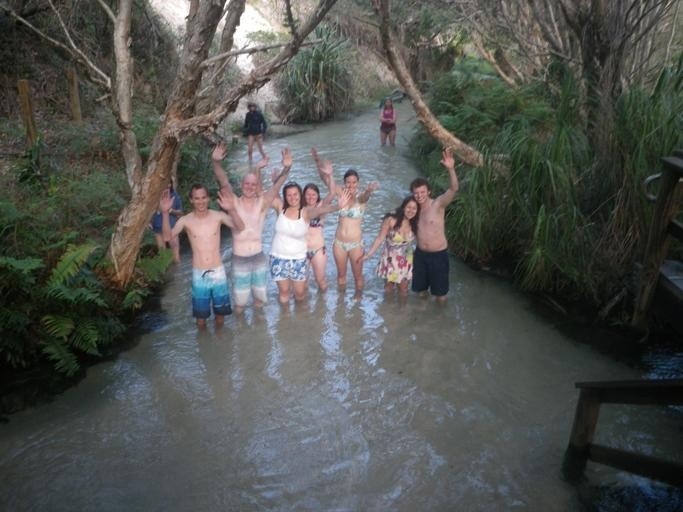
[241,102,267,159]
[159,183,246,327]
[255,153,353,305]
[409,149,459,300]
[270,157,337,292]
[146,178,184,265]
[356,196,421,296]
[310,146,379,300]
[378,97,397,145]
[212,141,293,313]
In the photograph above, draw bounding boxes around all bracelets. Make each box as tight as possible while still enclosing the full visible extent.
[364,253,370,258]
[168,208,173,214]
